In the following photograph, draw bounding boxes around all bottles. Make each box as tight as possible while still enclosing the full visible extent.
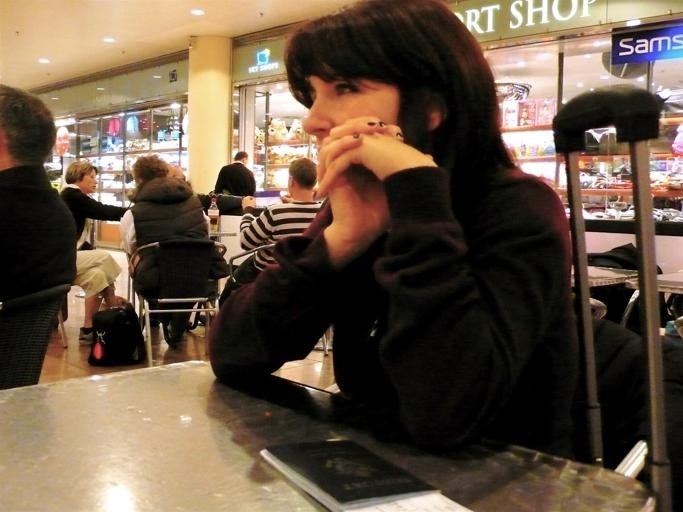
[207,198,219,233]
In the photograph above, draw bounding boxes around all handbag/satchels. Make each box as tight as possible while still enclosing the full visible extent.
[88,303,146,366]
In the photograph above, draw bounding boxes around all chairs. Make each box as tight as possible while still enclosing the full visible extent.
[125,238,226,368]
[228,239,330,356]
[0,283,70,389]
[218,215,255,266]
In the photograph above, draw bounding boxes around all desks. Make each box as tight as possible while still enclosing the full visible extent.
[571,260,682,328]
[0,360,655,512]
[209,231,237,238]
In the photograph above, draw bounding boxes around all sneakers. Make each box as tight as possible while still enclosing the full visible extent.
[79,327,93,344]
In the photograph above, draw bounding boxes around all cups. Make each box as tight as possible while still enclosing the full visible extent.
[279,191,290,204]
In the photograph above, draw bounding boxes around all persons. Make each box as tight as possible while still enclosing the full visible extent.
[60,160,132,345]
[0,82,78,306]
[120,156,212,349]
[199,156,324,326]
[214,151,256,196]
[164,163,242,212]
[209,0,581,458]
